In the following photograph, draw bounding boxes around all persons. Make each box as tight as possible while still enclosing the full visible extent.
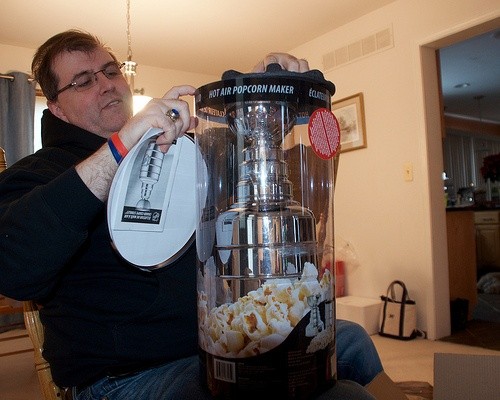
[0,29,405,400]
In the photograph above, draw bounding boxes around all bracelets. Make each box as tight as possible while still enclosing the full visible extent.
[108,133,129,166]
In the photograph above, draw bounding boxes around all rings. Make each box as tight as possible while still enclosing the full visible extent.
[165,109,179,121]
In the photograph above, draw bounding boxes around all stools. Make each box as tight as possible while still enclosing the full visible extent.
[336,296,385,335]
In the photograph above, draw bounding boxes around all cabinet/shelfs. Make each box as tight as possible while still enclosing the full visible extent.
[475,211,500,267]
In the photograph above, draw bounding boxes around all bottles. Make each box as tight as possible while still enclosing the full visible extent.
[135,140,165,212]
[194,62,339,400]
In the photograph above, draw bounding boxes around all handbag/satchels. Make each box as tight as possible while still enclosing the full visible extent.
[378,281,417,341]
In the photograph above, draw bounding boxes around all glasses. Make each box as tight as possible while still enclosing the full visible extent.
[55,62,126,97]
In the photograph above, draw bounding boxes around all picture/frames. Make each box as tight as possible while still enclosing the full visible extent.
[331,91,367,154]
